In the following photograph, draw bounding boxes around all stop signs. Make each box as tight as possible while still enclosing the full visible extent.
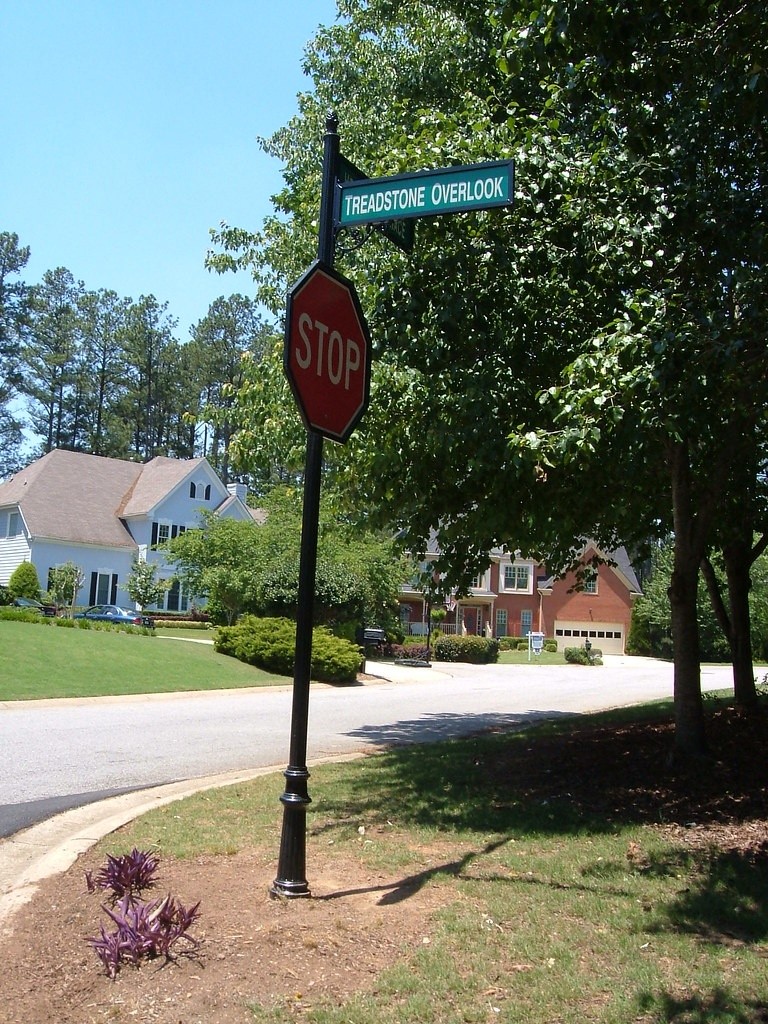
[278,263,373,443]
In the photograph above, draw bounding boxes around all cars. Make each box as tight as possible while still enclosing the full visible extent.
[60,604,157,630]
[4,596,56,619]
